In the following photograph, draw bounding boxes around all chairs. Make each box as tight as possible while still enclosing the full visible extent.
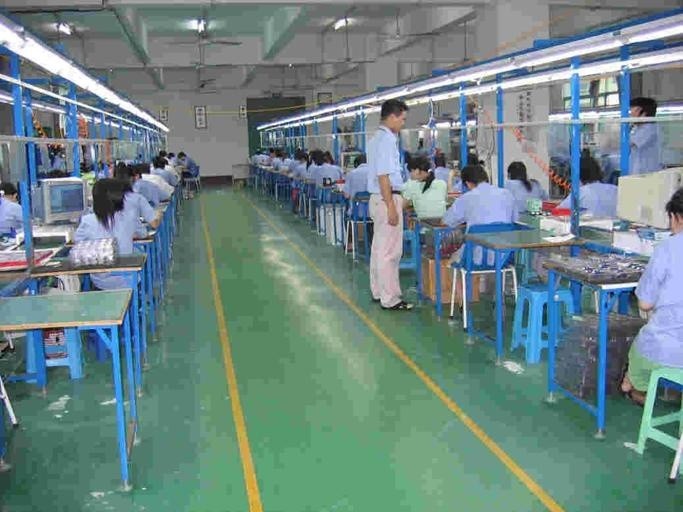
[184,165,201,192]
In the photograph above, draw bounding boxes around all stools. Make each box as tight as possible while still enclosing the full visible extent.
[634,369,683,475]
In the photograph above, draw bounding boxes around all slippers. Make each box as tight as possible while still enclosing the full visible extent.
[380,300,415,312]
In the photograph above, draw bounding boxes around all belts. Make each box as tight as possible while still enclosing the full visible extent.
[392,191,401,194]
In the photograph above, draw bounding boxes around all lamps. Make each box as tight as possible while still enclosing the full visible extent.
[0,14,172,134]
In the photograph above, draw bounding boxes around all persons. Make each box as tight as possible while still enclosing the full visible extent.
[617,187,683,406]
[246,146,621,313]
[0,150,194,288]
[629,98,660,174]
[368,99,414,311]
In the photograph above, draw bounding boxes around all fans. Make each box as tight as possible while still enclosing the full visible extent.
[172,10,241,71]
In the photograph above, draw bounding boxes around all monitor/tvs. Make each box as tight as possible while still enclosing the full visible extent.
[342,152,361,168]
[34,177,93,221]
[616,168,683,230]
